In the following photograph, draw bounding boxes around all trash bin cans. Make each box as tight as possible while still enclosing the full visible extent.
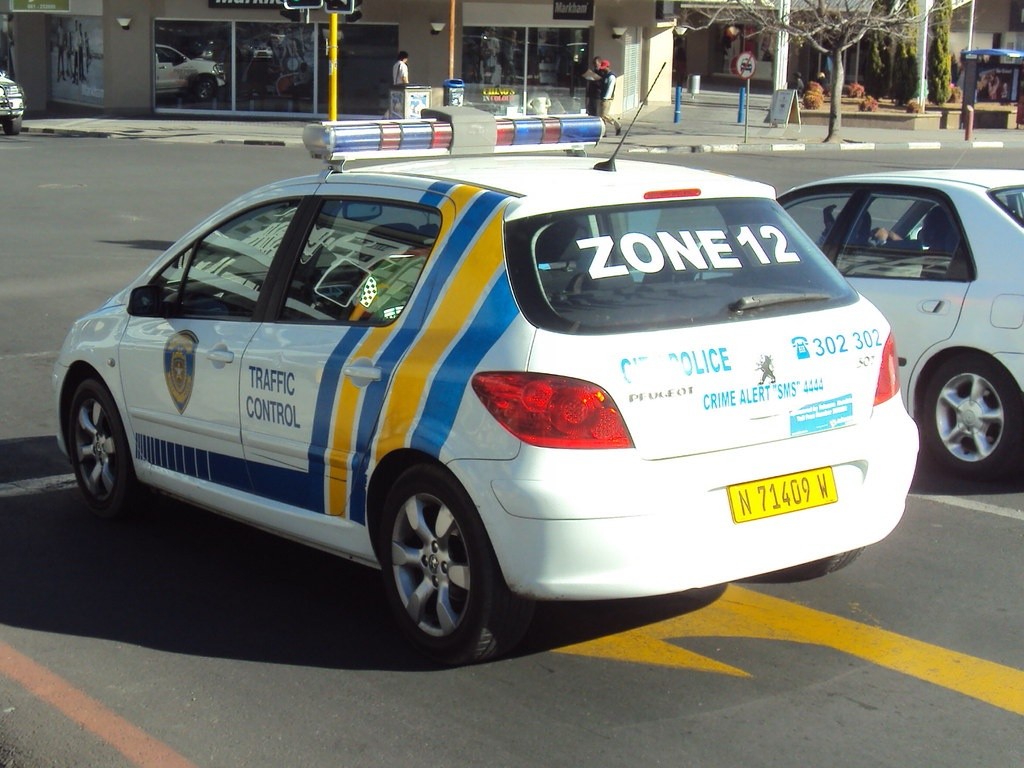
[441,79,465,107]
[387,82,433,119]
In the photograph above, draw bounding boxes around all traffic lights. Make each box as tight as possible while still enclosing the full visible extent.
[325,0,353,15]
[346,0,362,22]
[284,0,324,9]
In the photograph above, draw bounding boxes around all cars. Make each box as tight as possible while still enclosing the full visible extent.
[0,69,27,136]
[192,30,274,61]
[51,109,921,668]
[777,169,1024,483]
[155,43,226,102]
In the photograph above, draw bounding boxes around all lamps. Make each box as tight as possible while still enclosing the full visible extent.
[672,27,687,36]
[117,17,131,30]
[612,27,628,39]
[431,21,446,34]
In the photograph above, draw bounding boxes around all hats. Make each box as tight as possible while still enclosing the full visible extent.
[600,60,609,69]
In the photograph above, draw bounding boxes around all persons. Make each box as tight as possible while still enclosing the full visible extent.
[814,204,954,258]
[497,28,518,85]
[55,18,93,87]
[392,51,410,86]
[478,28,499,84]
[586,56,622,137]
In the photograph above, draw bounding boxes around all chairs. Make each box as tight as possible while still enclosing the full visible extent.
[641,257,697,282]
[356,223,425,285]
[535,224,598,292]
[846,209,872,245]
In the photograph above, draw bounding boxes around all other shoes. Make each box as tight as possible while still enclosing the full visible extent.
[616,126,622,135]
